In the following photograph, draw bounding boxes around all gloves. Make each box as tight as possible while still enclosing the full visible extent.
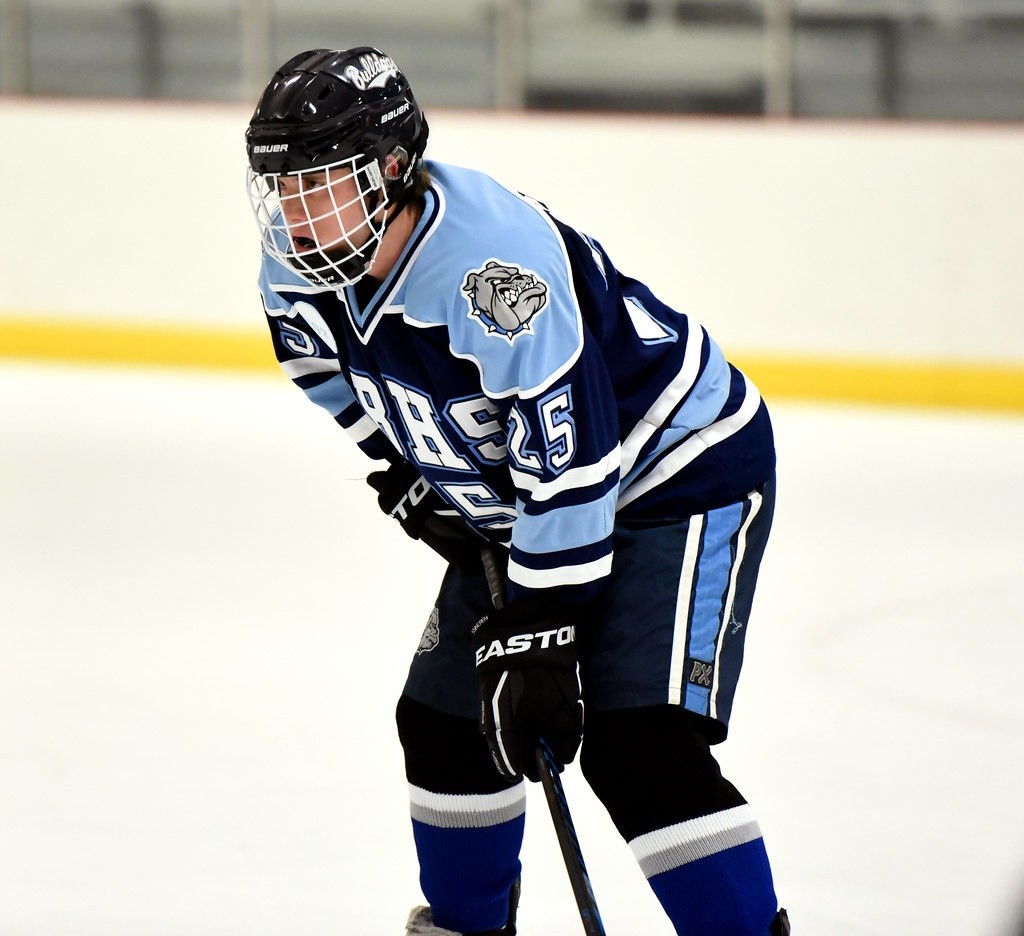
[460,596,585,784]
[366,461,481,576]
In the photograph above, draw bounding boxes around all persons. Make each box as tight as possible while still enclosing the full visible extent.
[245,46,791,936]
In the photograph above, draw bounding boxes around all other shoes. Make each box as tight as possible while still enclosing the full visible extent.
[405,872,520,936]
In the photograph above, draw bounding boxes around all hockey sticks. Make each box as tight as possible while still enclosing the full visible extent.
[480,543,607,936]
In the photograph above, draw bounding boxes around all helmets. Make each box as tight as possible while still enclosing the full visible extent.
[245,47,429,289]
